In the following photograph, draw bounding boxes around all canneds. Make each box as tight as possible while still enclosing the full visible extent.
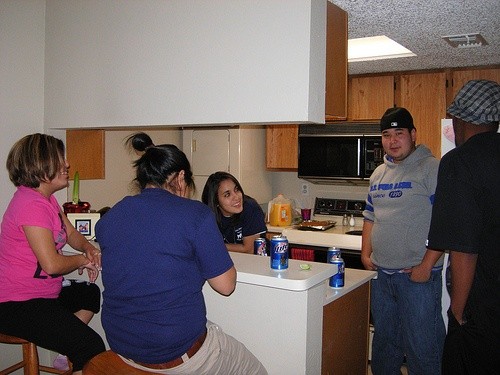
[254,237,266,257]
[327,247,345,289]
[270,236,289,270]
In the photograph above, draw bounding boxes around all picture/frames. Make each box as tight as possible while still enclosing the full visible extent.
[67,213,100,241]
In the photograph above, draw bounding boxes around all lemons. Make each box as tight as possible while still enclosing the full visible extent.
[300,264,310,269]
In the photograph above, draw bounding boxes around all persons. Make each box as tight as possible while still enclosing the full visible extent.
[426,80,500,375]
[0,134,107,375]
[95,133,271,375]
[362,107,445,375]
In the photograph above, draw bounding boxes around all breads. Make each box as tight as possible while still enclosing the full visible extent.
[301,220,330,226]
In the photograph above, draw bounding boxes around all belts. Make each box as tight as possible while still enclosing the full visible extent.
[123,328,207,370]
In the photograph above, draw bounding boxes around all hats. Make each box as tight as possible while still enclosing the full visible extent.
[380,107,413,131]
[448,79,500,125]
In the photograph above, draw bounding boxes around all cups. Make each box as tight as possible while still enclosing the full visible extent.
[302,209,311,219]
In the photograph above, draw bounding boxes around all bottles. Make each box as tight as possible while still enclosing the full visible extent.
[343,213,355,226]
[270,193,291,227]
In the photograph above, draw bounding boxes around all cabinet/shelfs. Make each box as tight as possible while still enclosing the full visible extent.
[266,64,500,169]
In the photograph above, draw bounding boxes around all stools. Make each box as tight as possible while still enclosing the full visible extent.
[0,336,40,375]
[82,350,158,375]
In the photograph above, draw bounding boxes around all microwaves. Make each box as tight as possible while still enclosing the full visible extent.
[298,124,386,186]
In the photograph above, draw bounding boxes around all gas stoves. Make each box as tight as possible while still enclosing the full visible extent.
[282,196,367,251]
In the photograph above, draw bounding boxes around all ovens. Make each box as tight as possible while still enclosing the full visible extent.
[287,243,366,270]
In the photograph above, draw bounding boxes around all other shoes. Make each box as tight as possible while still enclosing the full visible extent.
[53,354,70,370]
